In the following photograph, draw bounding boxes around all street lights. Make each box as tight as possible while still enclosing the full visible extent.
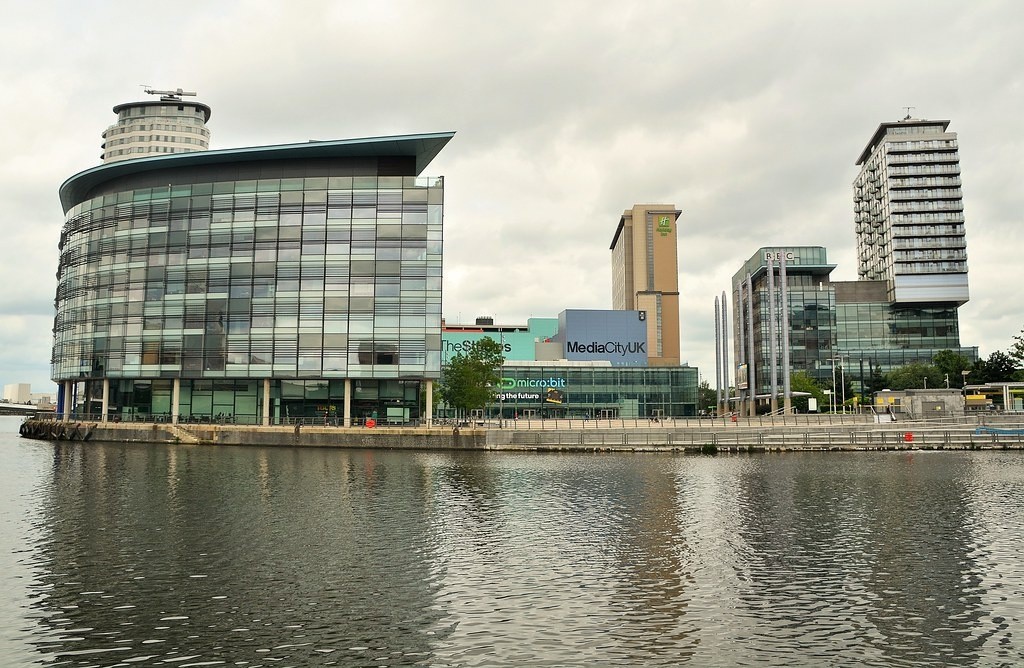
[834,355,850,414]
[826,358,842,414]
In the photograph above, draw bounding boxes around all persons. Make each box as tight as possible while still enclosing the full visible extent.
[498,413,500,421]
[738,370,747,385]
[543,413,546,421]
[596,411,601,420]
[128,408,231,423]
[324,411,329,426]
